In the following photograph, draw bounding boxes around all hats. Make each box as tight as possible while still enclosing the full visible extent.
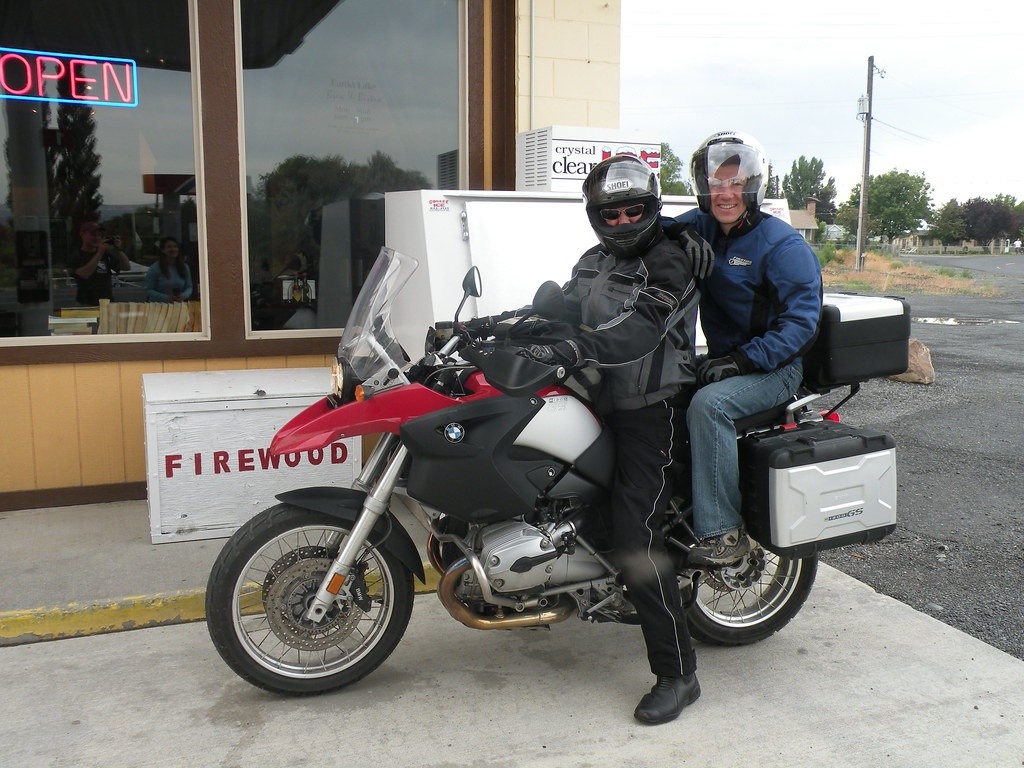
[81,222,107,233]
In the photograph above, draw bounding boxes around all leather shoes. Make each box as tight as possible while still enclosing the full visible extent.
[633,670,701,723]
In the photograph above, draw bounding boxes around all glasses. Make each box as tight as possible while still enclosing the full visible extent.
[600,205,645,221]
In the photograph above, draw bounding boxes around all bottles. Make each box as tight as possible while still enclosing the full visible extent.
[435,321,453,341]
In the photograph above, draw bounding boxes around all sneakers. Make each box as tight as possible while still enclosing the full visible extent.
[687,528,760,567]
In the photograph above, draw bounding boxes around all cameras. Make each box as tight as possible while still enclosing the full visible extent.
[105,236,115,246]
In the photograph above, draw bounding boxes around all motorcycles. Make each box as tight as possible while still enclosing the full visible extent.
[251,254,318,330]
[204,245,911,697]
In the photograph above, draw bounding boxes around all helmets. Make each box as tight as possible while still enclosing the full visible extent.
[582,155,662,258]
[691,131,768,212]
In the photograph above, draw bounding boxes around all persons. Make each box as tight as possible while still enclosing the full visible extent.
[433,154,703,726]
[1013,238,1023,256]
[68,224,131,307]
[144,237,195,303]
[658,130,824,568]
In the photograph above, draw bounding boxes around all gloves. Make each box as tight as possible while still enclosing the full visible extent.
[697,355,740,386]
[468,316,492,340]
[525,343,576,369]
[680,228,715,279]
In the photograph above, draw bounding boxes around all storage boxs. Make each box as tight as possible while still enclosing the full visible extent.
[142,367,362,545]
